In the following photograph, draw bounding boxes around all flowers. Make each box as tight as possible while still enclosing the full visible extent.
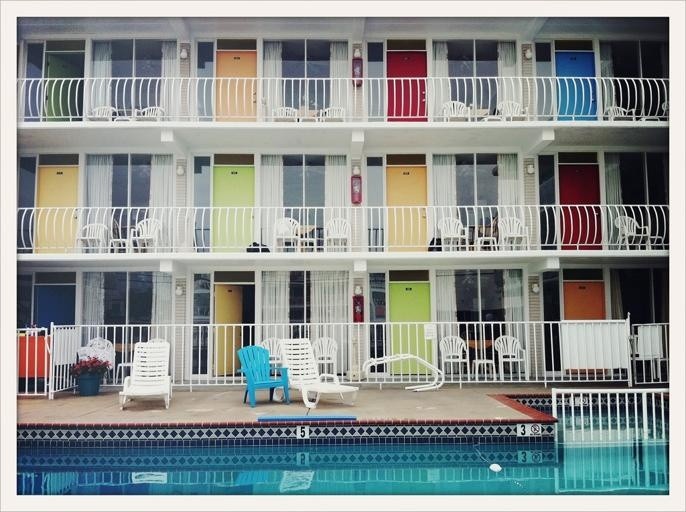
[72,357,111,375]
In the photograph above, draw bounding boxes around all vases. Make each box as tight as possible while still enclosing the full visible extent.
[77,369,99,396]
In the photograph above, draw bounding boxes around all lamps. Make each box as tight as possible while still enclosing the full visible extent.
[176,286,183,296]
[532,284,539,293]
[355,286,361,294]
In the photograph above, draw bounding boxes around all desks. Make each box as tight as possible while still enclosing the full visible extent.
[472,359,493,381]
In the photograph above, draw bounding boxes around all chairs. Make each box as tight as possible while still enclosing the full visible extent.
[439,336,467,381]
[273,217,301,253]
[324,218,352,252]
[497,102,525,121]
[604,106,635,121]
[498,217,530,251]
[130,218,162,252]
[134,107,165,122]
[443,102,470,122]
[272,107,298,122]
[119,340,171,410]
[614,215,652,251]
[437,217,469,252]
[77,224,113,252]
[320,107,346,122]
[89,106,119,122]
[495,335,525,381]
[236,337,359,409]
